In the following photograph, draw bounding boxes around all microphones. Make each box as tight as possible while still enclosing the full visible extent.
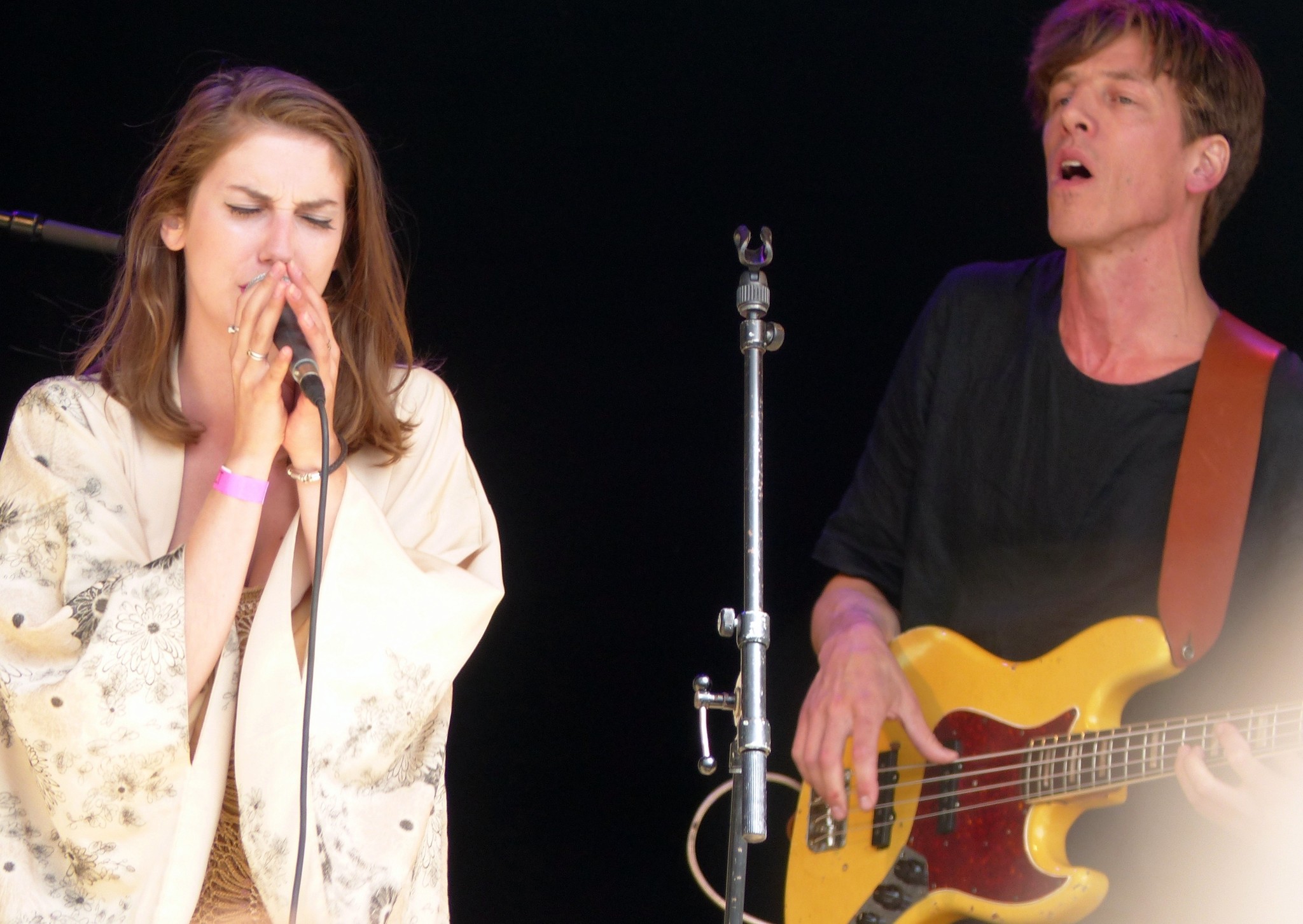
[245,272,327,407]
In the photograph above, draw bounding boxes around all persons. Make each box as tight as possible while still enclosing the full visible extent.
[790,1,1303,924]
[0,69,505,924]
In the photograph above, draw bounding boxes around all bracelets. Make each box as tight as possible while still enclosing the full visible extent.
[213,465,272,507]
[287,434,346,483]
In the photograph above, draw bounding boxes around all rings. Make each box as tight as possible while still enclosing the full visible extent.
[225,325,241,334]
[245,348,266,362]
[315,340,332,354]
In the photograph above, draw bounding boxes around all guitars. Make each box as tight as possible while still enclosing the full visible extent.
[783,617,1303,924]
